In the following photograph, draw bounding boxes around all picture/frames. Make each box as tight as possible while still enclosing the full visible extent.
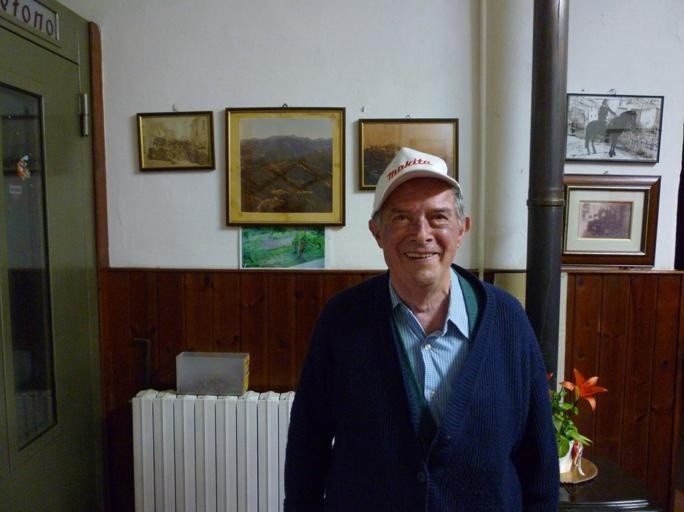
[225,106,346,227]
[136,110,216,173]
[565,93,665,163]
[559,173,662,266]
[358,118,460,190]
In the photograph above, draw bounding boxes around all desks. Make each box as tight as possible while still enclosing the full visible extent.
[557,455,651,512]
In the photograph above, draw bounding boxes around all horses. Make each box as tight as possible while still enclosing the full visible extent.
[584,110,638,157]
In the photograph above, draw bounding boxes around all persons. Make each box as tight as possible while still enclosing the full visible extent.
[279,147,559,510]
[598,99,617,137]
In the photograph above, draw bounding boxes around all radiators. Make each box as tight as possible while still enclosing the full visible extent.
[131,389,295,512]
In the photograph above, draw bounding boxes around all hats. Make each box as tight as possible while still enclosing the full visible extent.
[371,146,463,219]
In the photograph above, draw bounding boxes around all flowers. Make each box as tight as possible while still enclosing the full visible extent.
[546,367,608,457]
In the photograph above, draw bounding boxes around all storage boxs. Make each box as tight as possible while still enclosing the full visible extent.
[176,350,250,396]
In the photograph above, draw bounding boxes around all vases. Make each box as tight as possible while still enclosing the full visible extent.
[558,440,575,473]
